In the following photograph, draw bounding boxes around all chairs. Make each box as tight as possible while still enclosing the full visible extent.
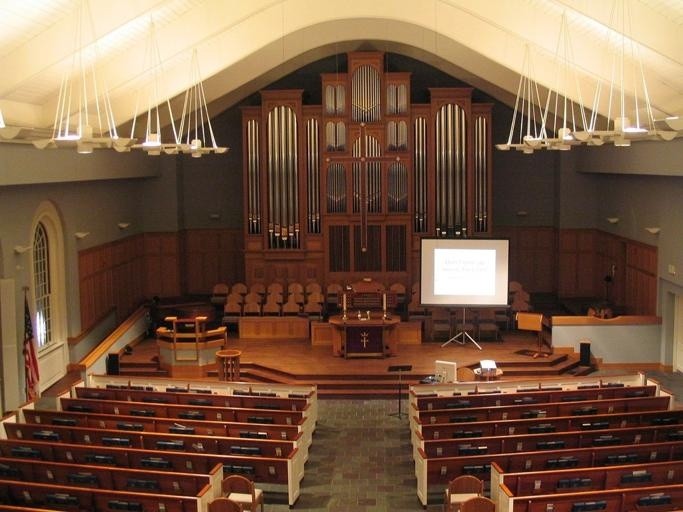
[213,278,529,339]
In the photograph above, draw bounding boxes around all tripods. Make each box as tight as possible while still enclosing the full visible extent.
[389,370,407,420]
[440,308,482,351]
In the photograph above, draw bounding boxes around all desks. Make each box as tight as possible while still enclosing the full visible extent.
[412,374,681,510]
[0,383,317,512]
[329,315,400,359]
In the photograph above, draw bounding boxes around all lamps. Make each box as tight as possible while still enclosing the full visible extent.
[33,1,230,158]
[493,1,682,154]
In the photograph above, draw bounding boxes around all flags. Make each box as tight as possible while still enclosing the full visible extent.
[23,292,40,403]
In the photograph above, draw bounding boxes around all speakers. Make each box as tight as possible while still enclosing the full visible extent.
[580,343,591,366]
[109,353,119,375]
